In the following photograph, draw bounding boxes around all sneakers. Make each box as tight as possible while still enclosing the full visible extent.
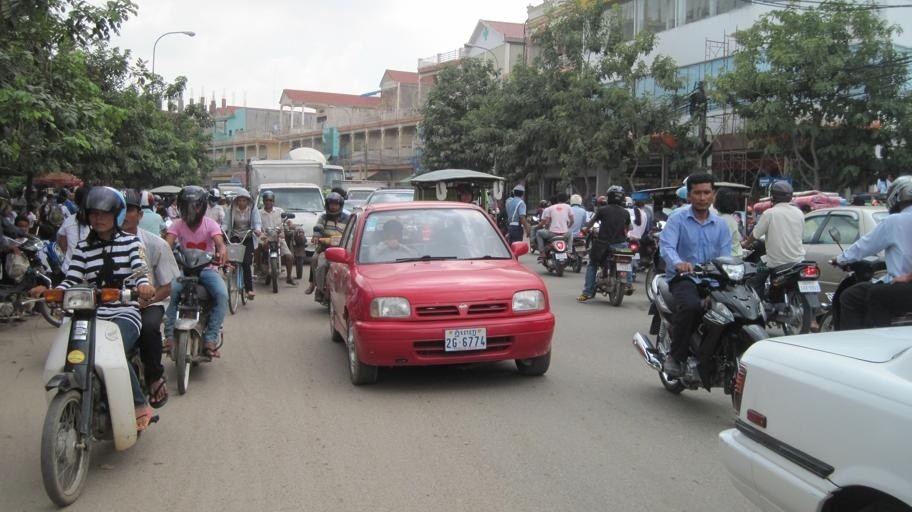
[577,295,593,300]
[663,351,681,377]
[305,286,315,294]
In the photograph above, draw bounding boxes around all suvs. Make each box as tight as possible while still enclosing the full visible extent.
[756,205,900,294]
[746,185,847,219]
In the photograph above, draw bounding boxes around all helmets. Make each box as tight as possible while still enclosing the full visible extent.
[262,191,274,203]
[539,199,547,208]
[323,192,344,216]
[606,184,624,204]
[769,179,793,203]
[174,186,208,231]
[569,194,582,206]
[210,187,221,207]
[885,176,912,213]
[76,186,155,232]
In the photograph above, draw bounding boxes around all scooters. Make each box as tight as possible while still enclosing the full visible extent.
[161,248,236,394]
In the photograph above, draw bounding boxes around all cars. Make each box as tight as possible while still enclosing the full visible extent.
[714,317,912,512]
[320,199,557,385]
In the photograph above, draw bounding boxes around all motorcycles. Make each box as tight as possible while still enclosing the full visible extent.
[308,219,346,310]
[812,223,912,333]
[528,191,824,416]
[21,268,160,508]
[1,181,303,329]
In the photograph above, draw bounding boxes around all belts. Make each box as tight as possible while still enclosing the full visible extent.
[509,222,522,226]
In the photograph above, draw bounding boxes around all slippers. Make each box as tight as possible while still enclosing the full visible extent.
[148,377,168,408]
[203,347,219,358]
[136,409,152,431]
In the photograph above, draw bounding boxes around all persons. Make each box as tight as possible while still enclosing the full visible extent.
[30,188,157,428]
[139,185,295,358]
[506,184,530,262]
[304,187,354,294]
[829,173,911,331]
[456,184,476,205]
[368,220,415,261]
[1,184,93,323]
[659,172,731,375]
[106,190,180,410]
[712,179,812,303]
[534,176,690,301]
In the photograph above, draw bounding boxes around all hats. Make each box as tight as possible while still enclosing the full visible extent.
[513,184,525,191]
[675,186,687,200]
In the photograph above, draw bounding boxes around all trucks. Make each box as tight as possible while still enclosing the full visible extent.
[248,158,523,279]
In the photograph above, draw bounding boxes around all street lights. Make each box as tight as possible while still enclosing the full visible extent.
[149,27,202,110]
[461,42,504,89]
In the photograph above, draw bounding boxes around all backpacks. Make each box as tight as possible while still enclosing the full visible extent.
[44,240,64,267]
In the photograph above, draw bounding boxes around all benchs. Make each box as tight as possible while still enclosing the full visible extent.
[359,230,477,261]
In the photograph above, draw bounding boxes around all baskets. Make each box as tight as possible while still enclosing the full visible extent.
[226,243,246,263]
